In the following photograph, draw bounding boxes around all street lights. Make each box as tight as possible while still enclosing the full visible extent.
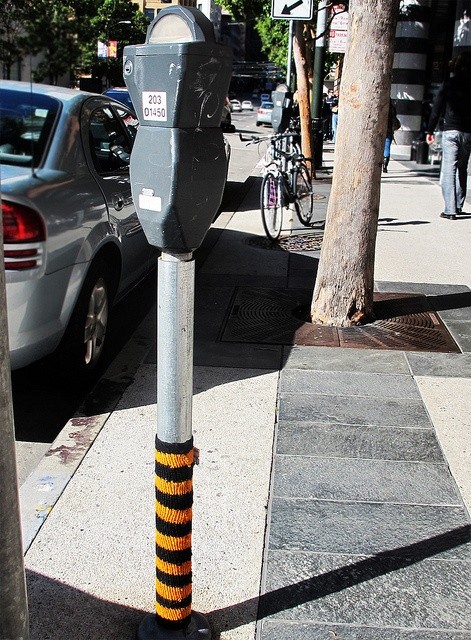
[101,20,131,69]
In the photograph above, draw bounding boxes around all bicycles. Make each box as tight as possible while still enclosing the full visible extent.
[238,133,314,242]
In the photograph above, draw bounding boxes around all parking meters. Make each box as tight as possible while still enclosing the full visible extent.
[271,84,292,229]
[122,4,233,640]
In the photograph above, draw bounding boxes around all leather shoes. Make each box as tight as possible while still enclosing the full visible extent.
[440,212,456,220]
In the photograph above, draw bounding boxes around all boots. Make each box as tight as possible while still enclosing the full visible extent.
[383,157,389,173]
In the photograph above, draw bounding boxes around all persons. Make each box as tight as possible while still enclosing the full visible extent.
[323,89,339,144]
[383,97,396,175]
[426,53,471,220]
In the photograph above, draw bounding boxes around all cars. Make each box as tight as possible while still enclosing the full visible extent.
[102,86,134,111]
[256,102,274,126]
[241,101,253,111]
[0,79,160,393]
[229,99,242,112]
